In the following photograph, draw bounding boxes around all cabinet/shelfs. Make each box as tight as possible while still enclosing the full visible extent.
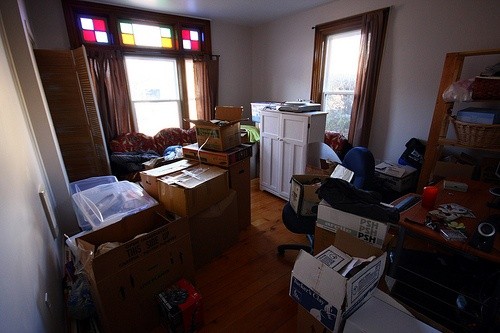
[259,109,328,201]
[417,48,500,195]
[33,46,112,182]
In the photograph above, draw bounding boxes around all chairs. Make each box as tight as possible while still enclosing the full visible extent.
[277,146,375,257]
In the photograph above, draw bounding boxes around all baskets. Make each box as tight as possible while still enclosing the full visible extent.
[450,116,500,148]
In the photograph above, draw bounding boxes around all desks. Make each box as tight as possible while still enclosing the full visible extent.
[399,176,500,263]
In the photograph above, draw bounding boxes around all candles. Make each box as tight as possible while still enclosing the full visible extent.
[422,186,438,209]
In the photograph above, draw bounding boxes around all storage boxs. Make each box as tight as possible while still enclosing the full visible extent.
[66,105,474,333]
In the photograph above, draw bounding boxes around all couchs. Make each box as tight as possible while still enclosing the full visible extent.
[110,118,254,179]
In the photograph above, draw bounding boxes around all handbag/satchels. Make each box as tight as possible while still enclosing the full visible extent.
[315,177,400,223]
